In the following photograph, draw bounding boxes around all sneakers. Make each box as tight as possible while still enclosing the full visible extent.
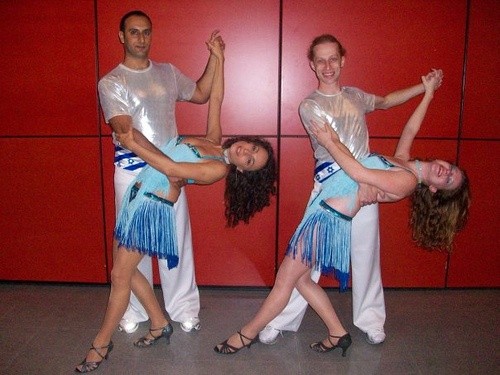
[178,315,202,333]
[258,324,283,346]
[364,326,386,345]
[116,318,139,334]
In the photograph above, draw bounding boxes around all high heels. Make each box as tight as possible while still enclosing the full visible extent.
[308,332,352,356]
[76,339,114,374]
[132,322,173,348]
[214,329,260,355]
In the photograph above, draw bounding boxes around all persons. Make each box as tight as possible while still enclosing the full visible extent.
[259,34,444,345]
[96,9,226,334]
[213,66,472,357]
[75,35,277,374]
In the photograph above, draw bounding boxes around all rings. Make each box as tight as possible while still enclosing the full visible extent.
[118,142,120,146]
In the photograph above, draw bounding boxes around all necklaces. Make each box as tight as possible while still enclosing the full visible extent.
[416,160,422,184]
[224,149,231,165]
